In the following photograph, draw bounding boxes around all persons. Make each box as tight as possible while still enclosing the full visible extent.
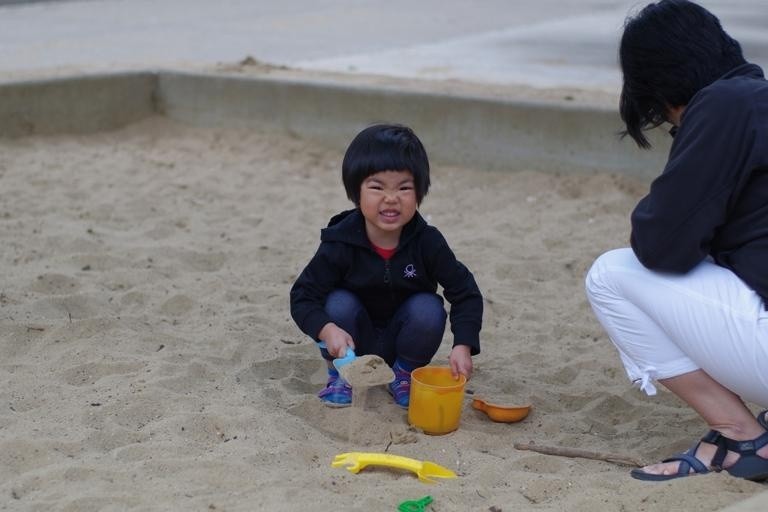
[288,123,484,409]
[586,2,768,481]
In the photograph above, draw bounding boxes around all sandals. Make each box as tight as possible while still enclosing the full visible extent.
[629,409,768,484]
[319,368,352,408]
[388,357,414,408]
